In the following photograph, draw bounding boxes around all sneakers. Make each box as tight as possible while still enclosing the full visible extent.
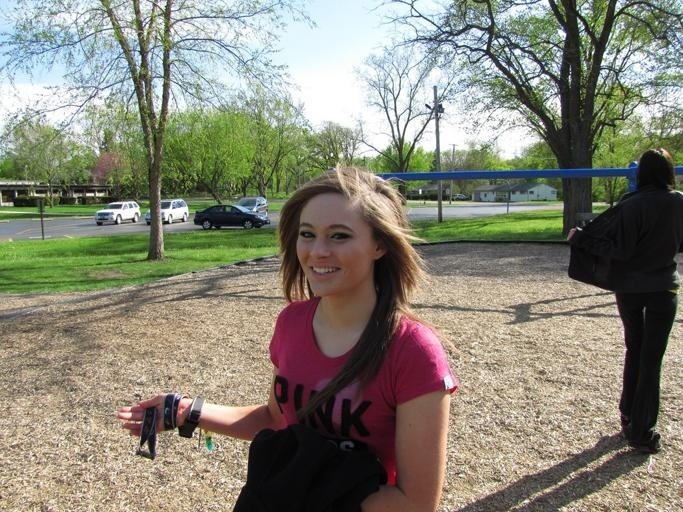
[621,415,663,453]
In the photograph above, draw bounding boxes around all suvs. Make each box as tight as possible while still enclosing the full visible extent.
[94,201,140,225]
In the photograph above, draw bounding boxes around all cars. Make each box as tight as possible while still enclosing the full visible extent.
[193,203,269,230]
[144,198,189,224]
[235,197,268,217]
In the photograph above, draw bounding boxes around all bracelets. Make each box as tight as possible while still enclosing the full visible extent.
[178,395,206,440]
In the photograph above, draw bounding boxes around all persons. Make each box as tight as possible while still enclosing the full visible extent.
[566,148,683,452]
[117,165,461,512]
